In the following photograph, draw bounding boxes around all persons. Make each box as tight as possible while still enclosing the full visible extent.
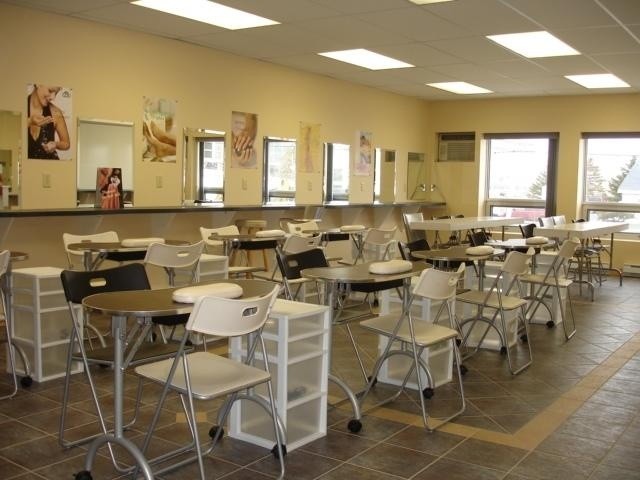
[101,170,121,208]
[233,114,257,164]
[28,84,70,160]
[144,121,176,157]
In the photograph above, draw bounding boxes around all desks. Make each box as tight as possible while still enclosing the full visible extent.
[300,259,424,432]
[74,279,283,478]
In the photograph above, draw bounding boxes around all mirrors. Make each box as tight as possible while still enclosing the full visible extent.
[74,114,138,210]
[261,134,299,208]
[0,108,25,211]
[180,125,229,210]
[405,151,432,205]
[321,141,353,208]
[371,146,399,206]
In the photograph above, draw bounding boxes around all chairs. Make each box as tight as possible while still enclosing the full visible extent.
[58,262,196,472]
[0,214,630,399]
[353,262,466,432]
[134,285,286,480]
